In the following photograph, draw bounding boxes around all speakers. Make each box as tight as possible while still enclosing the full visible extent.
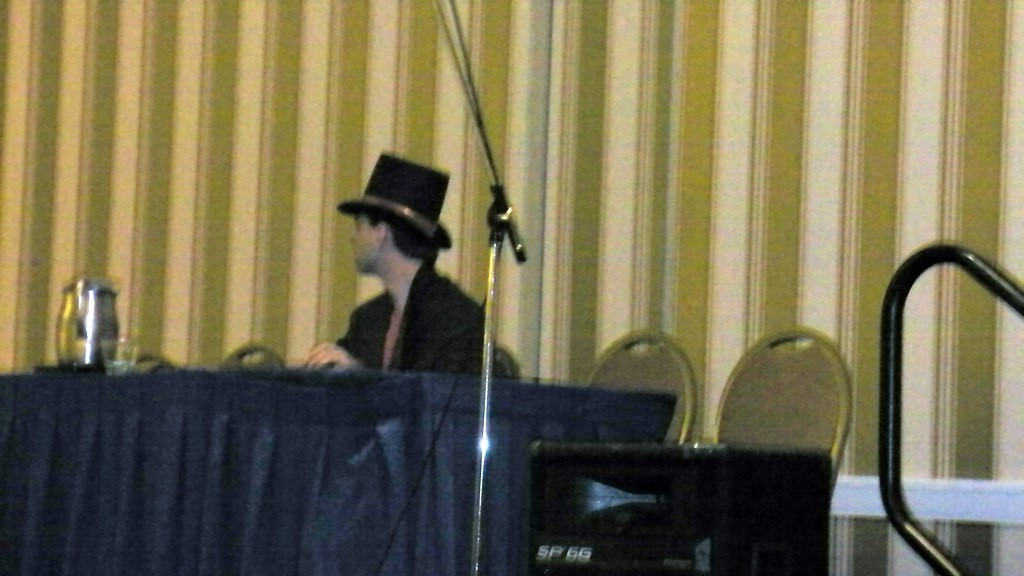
[534,447,834,576]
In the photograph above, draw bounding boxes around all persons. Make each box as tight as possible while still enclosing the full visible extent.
[299,153,514,375]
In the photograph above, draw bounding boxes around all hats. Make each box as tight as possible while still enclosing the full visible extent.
[338,154,451,248]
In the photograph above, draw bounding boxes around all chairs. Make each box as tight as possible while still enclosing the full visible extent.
[584,330,704,440]
[717,324,856,488]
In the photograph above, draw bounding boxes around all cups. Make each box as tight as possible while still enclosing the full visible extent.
[56,280,117,367]
[100,339,137,374]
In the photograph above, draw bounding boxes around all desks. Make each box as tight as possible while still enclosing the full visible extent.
[0,365,679,576]
